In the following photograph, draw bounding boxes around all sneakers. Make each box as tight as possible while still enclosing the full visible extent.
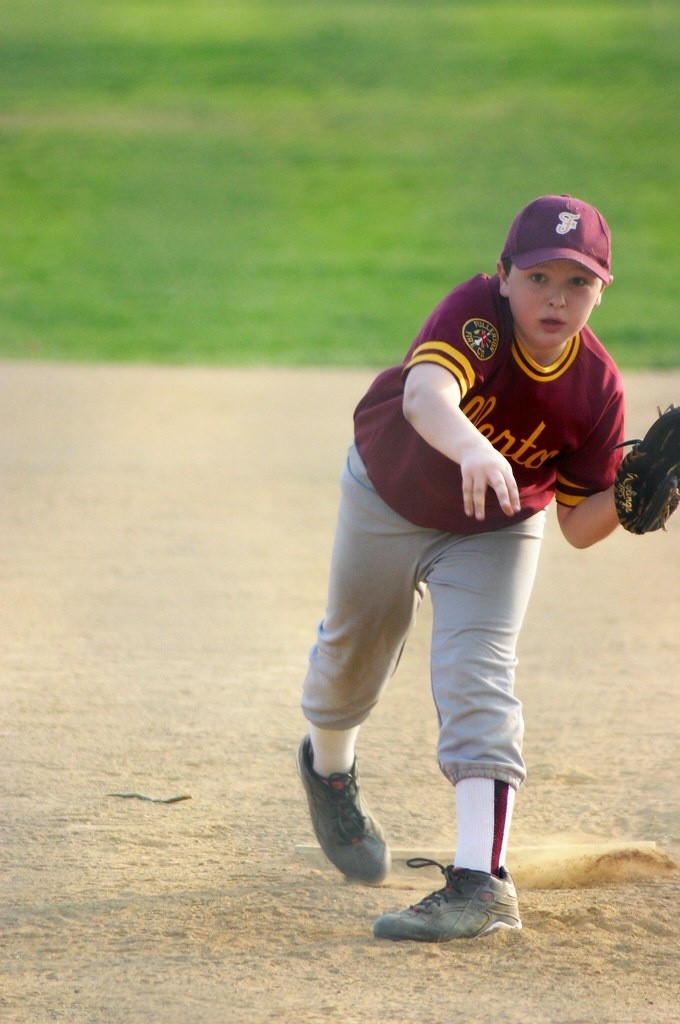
[296,734,391,887]
[372,857,522,943]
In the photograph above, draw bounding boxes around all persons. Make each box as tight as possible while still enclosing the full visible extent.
[297,195,680,944]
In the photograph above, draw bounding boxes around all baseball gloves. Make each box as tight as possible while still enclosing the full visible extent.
[610,402,680,536]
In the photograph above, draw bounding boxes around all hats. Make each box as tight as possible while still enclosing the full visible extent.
[500,194,611,285]
